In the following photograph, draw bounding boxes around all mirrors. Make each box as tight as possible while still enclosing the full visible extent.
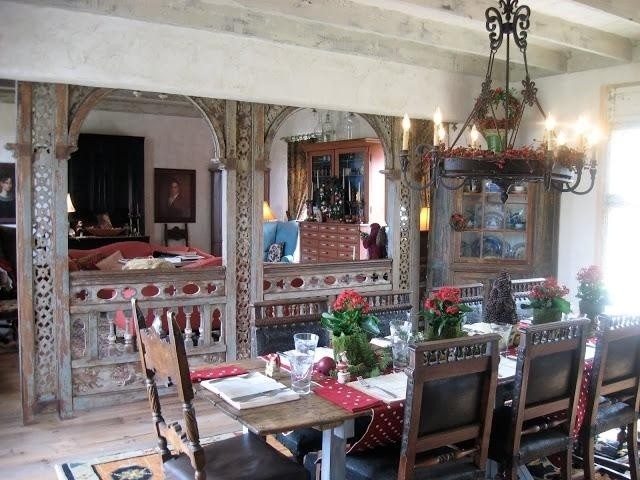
[67,133,146,234]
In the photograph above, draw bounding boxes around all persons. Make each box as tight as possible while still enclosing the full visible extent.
[0,176,12,198]
[167,181,183,208]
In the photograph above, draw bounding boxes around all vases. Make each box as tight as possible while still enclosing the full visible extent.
[485,129,507,152]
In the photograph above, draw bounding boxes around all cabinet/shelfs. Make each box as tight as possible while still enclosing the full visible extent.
[284,136,386,265]
[424,151,561,290]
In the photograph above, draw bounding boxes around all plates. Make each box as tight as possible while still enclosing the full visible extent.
[344,371,410,404]
[462,180,528,261]
[498,354,517,378]
[281,346,334,366]
[369,334,391,348]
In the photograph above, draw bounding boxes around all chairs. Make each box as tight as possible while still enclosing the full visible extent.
[263,221,299,265]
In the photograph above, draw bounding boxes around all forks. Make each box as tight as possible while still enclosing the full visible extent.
[356,375,397,400]
[208,371,256,386]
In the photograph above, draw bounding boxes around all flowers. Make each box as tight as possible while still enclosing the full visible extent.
[474,88,522,130]
[321,263,612,338]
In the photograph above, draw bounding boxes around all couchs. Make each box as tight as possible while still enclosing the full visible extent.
[69,239,225,350]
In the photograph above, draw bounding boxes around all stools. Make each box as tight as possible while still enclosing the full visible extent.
[0,299,18,350]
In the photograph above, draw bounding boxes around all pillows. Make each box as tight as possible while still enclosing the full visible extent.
[68,252,127,272]
[266,242,285,262]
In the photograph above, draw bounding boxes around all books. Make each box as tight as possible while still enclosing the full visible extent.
[150,249,206,263]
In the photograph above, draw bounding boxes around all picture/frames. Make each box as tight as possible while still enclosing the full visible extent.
[153,166,196,224]
[601,80,640,131]
[0,162,16,224]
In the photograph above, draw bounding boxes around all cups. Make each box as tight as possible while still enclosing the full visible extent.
[489,323,514,352]
[289,352,314,396]
[389,319,411,373]
[293,333,322,352]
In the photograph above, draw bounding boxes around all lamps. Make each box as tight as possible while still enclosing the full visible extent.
[419,207,430,232]
[395,0,603,202]
[262,200,277,222]
[67,194,77,234]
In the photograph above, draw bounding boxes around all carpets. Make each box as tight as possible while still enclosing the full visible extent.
[54,427,294,480]
